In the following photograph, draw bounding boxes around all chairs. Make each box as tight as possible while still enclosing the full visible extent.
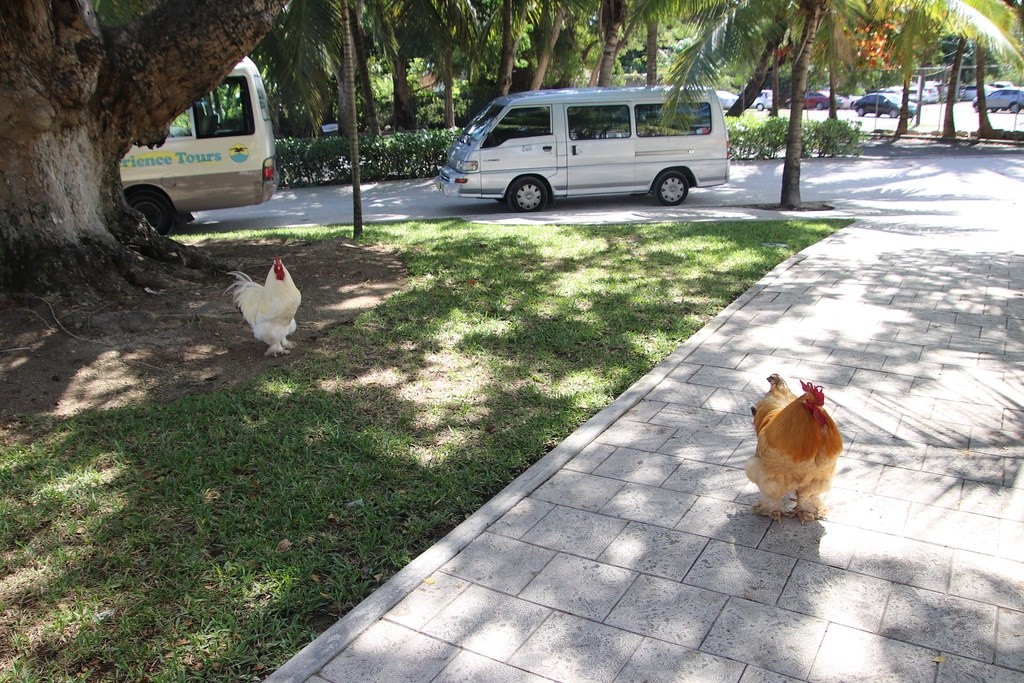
[190,101,215,139]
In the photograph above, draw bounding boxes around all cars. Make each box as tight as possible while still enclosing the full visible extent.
[715,90,739,109]
[850,92,918,118]
[868,81,940,103]
[785,90,843,110]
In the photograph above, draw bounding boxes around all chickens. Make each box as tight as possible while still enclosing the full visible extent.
[223,254,301,358]
[743,373,844,526]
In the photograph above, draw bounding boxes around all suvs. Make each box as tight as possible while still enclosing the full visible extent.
[973,89,1024,113]
[958,81,1014,101]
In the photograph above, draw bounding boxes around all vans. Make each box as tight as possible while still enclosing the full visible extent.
[435,84,731,213]
[748,90,773,111]
[120,55,280,237]
